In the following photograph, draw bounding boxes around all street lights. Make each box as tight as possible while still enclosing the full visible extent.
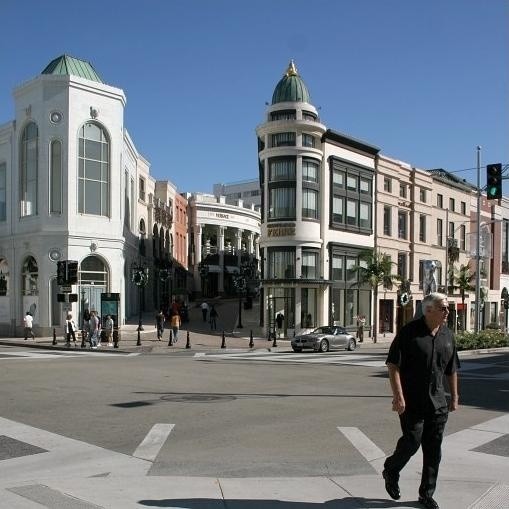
[233,274,245,329]
[129,255,151,331]
[199,259,209,303]
[159,266,171,309]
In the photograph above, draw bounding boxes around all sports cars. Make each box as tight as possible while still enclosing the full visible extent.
[291,326,356,351]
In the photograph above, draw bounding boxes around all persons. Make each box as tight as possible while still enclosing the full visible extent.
[381,292,462,509]
[27,313,38,338]
[65,301,219,349]
[357,315,366,342]
[275,312,284,338]
[23,312,35,340]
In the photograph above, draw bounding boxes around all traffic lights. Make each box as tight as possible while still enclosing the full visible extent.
[70,261,78,282]
[486,164,503,200]
[57,262,65,282]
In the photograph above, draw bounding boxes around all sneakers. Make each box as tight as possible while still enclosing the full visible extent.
[69,342,113,349]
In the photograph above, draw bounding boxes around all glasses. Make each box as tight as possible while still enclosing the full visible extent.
[428,306,450,312]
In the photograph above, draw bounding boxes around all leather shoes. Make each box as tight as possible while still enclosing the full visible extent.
[418,495,438,508]
[382,469,401,501]
